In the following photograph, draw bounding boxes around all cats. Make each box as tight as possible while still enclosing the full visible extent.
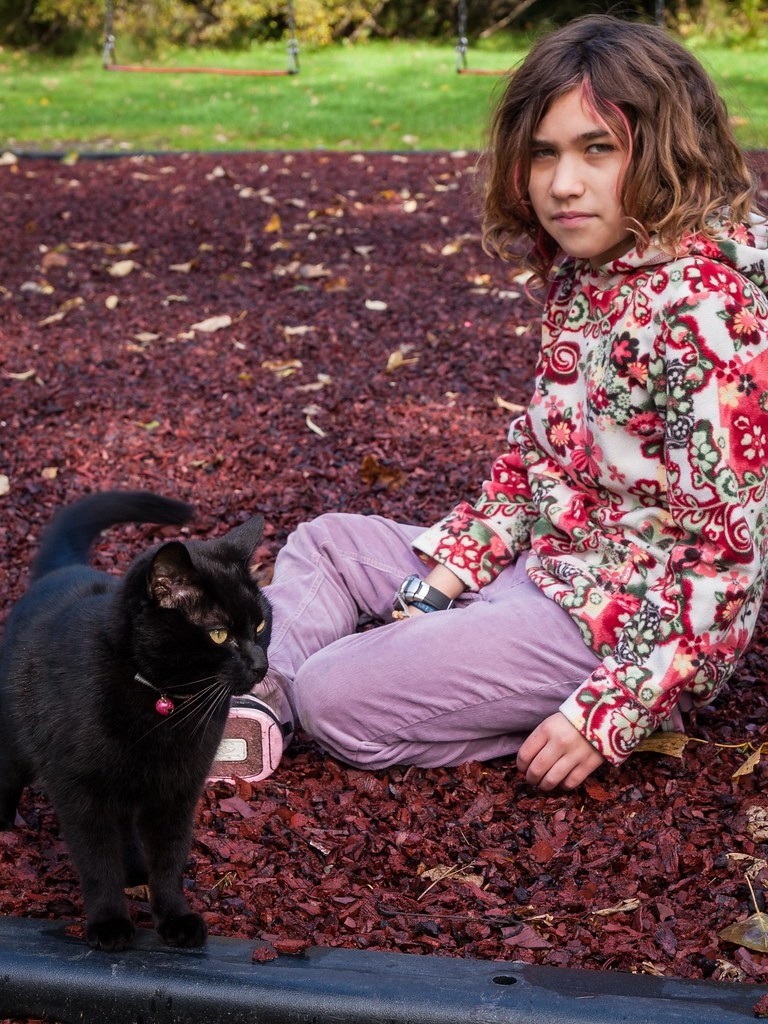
[0,490,274,954]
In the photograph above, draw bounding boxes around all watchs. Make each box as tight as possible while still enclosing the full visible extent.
[392,574,456,610]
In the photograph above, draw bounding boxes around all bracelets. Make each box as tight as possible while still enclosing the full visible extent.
[391,601,439,621]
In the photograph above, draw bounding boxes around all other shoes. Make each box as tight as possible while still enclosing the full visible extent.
[204,694,294,783]
[651,704,685,737]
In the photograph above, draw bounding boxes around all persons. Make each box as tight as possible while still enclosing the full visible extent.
[204,14,768,790]
